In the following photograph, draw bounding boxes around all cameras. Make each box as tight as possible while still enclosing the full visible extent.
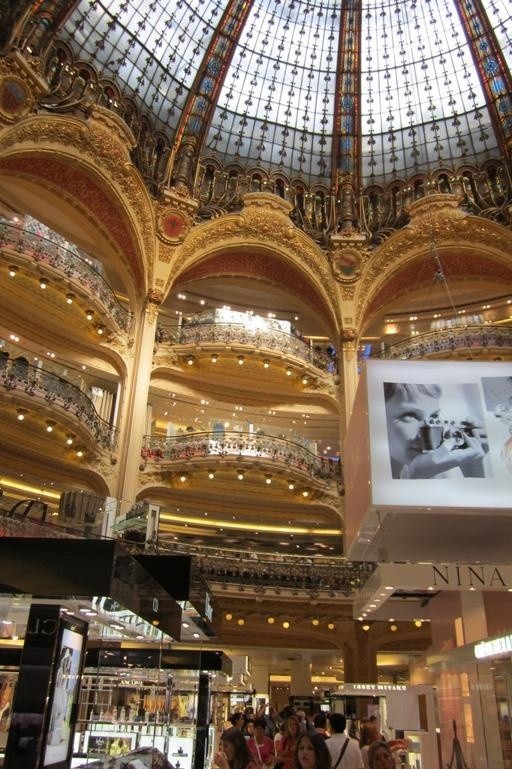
[424,417,473,450]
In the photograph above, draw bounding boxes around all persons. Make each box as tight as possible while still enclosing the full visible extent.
[383,380,489,480]
[208,319,215,324]
[337,183,361,235]
[48,646,73,749]
[171,143,197,195]
[31,29,43,51]
[291,323,336,374]
[214,698,396,769]
[445,717,470,769]
[162,695,181,720]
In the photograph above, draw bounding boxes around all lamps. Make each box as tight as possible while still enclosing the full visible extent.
[224,609,424,632]
[11,406,86,459]
[183,350,311,385]
[7,264,109,338]
[176,468,311,498]
